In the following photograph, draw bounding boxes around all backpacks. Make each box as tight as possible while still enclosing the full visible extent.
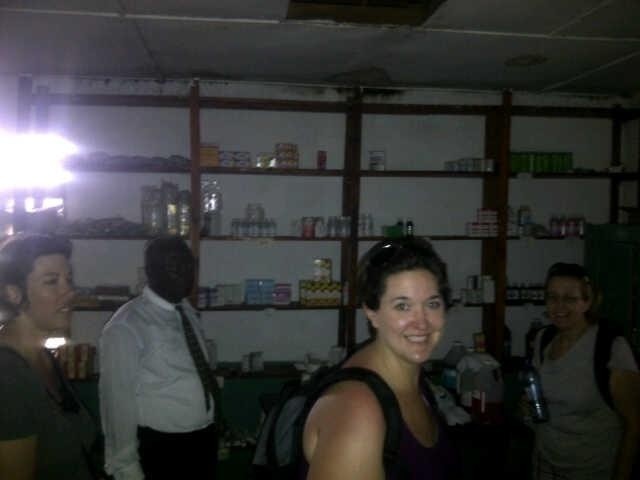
[249,364,401,479]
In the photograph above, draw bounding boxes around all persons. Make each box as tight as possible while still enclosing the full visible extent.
[288,236,452,480]
[517,260,640,480]
[0,232,103,480]
[96,234,224,480]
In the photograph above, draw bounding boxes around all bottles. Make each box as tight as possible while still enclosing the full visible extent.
[407,216,413,233]
[178,191,193,236]
[504,278,542,305]
[518,203,586,238]
[229,205,373,242]
[444,340,470,398]
[523,363,547,424]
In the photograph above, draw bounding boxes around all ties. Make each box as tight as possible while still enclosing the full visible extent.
[176,305,222,424]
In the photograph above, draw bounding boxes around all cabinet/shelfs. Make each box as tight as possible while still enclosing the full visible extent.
[1,74,639,480]
